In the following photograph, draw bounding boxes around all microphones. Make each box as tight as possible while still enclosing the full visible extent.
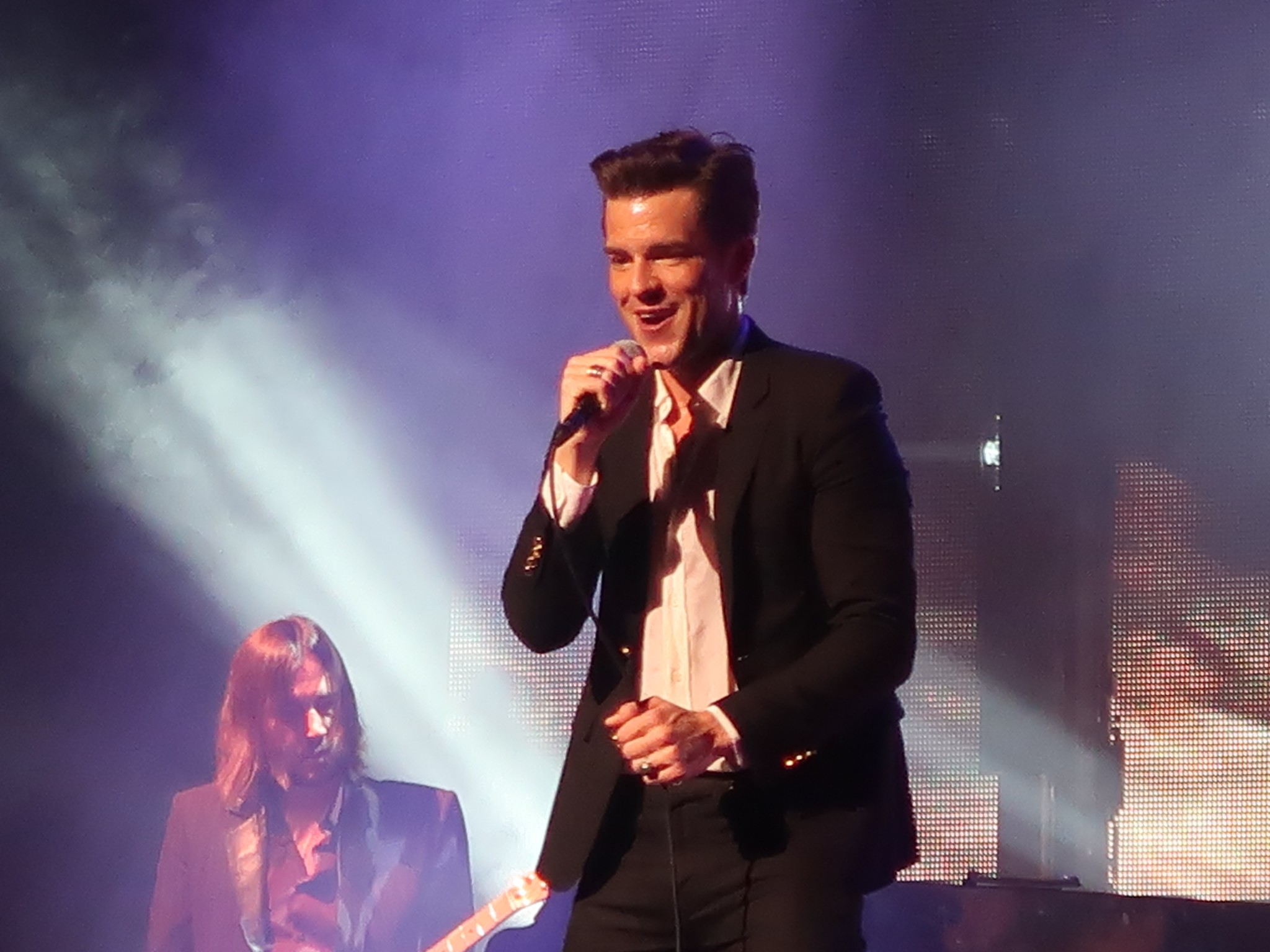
[552,340,647,444]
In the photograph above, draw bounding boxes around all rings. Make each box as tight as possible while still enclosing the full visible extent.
[639,758,656,775]
[611,729,619,747]
[588,365,605,379]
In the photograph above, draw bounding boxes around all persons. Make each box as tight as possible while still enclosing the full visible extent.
[146,617,476,952]
[501,128,917,952]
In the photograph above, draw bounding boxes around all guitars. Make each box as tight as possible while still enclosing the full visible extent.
[422,874,549,952]
